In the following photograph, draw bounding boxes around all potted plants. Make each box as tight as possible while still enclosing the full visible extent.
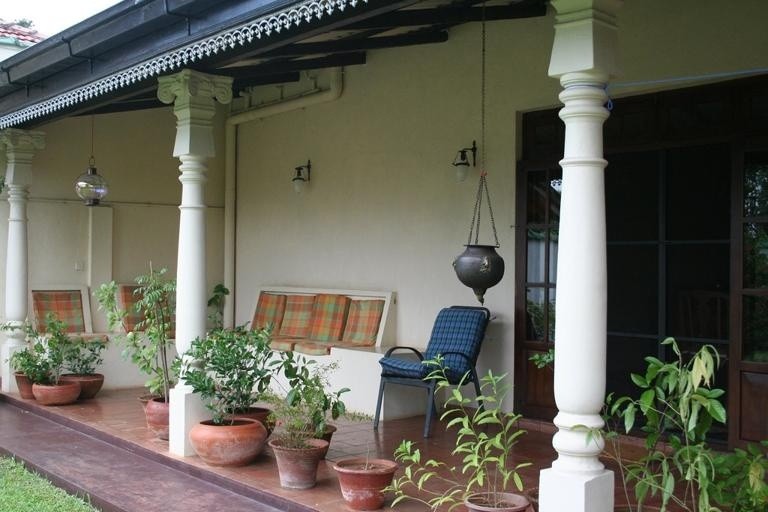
[0,321,105,409]
[381,358,530,512]
[269,357,337,495]
[96,271,177,442]
[189,284,274,464]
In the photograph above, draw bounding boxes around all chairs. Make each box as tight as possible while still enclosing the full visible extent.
[371,305,493,438]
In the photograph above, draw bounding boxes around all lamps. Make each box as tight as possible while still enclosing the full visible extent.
[74,115,110,207]
[293,155,312,199]
[450,137,477,183]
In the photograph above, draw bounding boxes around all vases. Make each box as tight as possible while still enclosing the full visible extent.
[335,458,401,510]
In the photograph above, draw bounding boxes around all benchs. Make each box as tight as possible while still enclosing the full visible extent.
[32,287,179,387]
[248,289,451,423]
